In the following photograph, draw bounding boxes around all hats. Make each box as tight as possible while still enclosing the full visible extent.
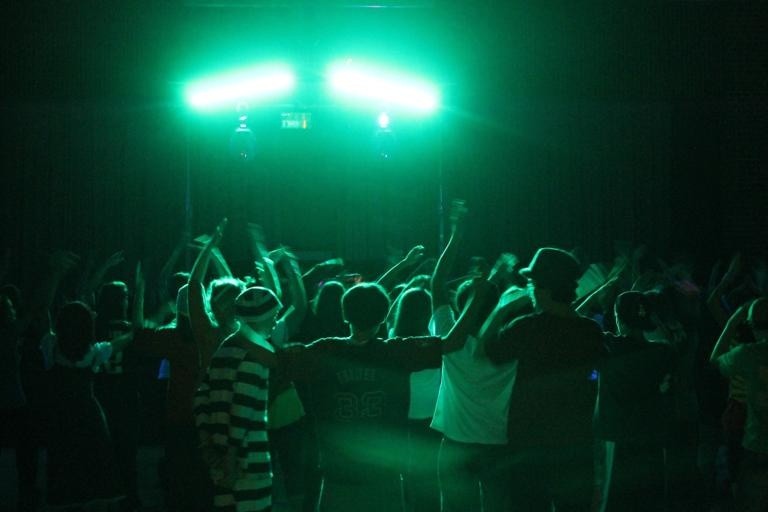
[615,289,658,331]
[233,286,283,322]
[518,247,581,288]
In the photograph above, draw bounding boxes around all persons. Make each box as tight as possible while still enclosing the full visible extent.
[1,203,768,512]
